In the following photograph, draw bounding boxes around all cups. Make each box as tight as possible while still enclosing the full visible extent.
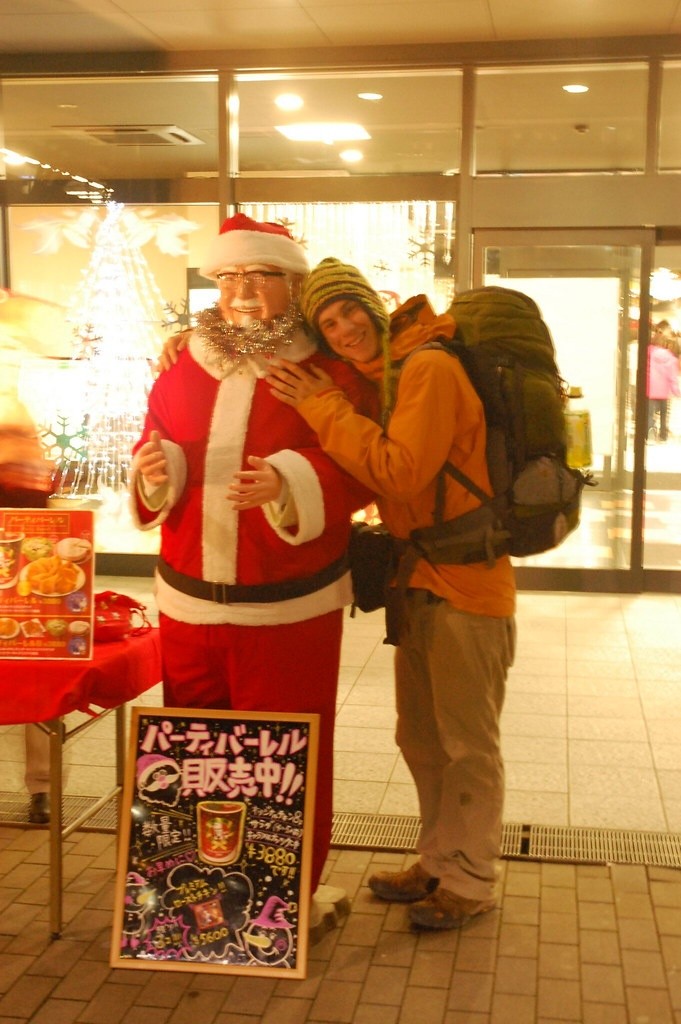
[0,533,25,589]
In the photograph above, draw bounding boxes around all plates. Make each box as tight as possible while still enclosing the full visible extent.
[20,557,86,597]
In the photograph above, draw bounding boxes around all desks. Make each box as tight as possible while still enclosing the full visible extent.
[0,628,164,938]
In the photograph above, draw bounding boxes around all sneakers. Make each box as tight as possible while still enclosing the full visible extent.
[368,861,442,901]
[407,888,497,929]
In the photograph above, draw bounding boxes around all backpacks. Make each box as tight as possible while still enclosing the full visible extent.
[398,285,597,566]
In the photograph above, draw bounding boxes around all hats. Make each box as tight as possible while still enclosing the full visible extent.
[299,258,395,437]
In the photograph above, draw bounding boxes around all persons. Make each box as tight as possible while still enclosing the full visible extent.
[263,257,516,930]
[130,213,380,910]
[626,321,680,441]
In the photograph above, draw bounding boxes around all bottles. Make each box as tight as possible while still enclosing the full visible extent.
[351,520,386,611]
[565,386,592,470]
